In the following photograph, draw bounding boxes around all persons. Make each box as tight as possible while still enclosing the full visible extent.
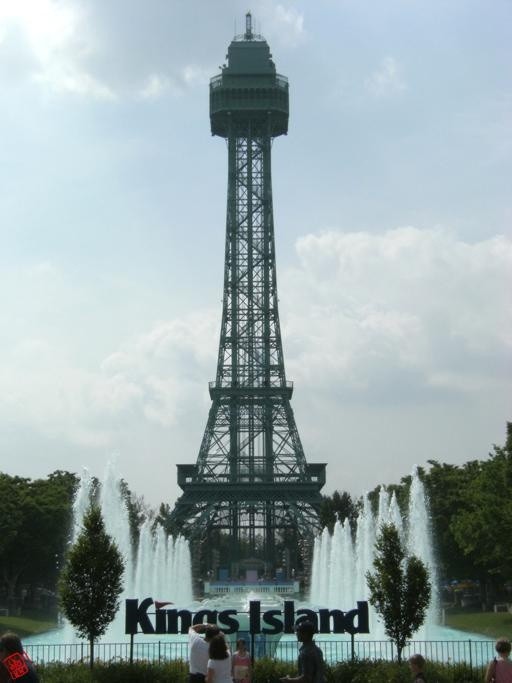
[205,628,234,682]
[408,653,429,682]
[278,619,323,682]
[230,636,253,682]
[484,638,511,682]
[187,622,221,683]
[0,632,40,682]
